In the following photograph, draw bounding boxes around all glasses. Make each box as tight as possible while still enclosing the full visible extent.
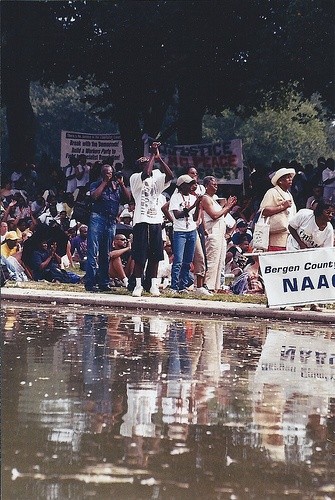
[119,239,129,241]
[191,172,198,175]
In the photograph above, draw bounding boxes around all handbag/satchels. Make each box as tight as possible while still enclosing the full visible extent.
[254,208,271,250]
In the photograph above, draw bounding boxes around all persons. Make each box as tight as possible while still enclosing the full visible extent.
[130,142,174,297]
[31,236,82,283]
[286,201,334,312]
[85,165,130,292]
[260,168,305,308]
[1,153,335,296]
[201,176,237,294]
[75,315,335,467]
[168,175,203,294]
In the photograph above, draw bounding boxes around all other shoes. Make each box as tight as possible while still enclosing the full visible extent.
[100,285,111,291]
[197,287,213,296]
[133,286,143,296]
[85,285,99,293]
[150,287,160,296]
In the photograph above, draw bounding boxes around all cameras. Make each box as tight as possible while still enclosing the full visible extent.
[110,172,123,181]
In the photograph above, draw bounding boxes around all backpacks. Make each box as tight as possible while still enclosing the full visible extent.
[232,272,251,294]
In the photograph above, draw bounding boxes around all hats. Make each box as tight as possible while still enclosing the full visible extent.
[237,221,249,228]
[5,231,20,241]
[271,167,296,187]
[176,174,196,186]
[135,157,150,163]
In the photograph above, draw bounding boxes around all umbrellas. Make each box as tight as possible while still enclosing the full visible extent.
[22,226,68,270]
